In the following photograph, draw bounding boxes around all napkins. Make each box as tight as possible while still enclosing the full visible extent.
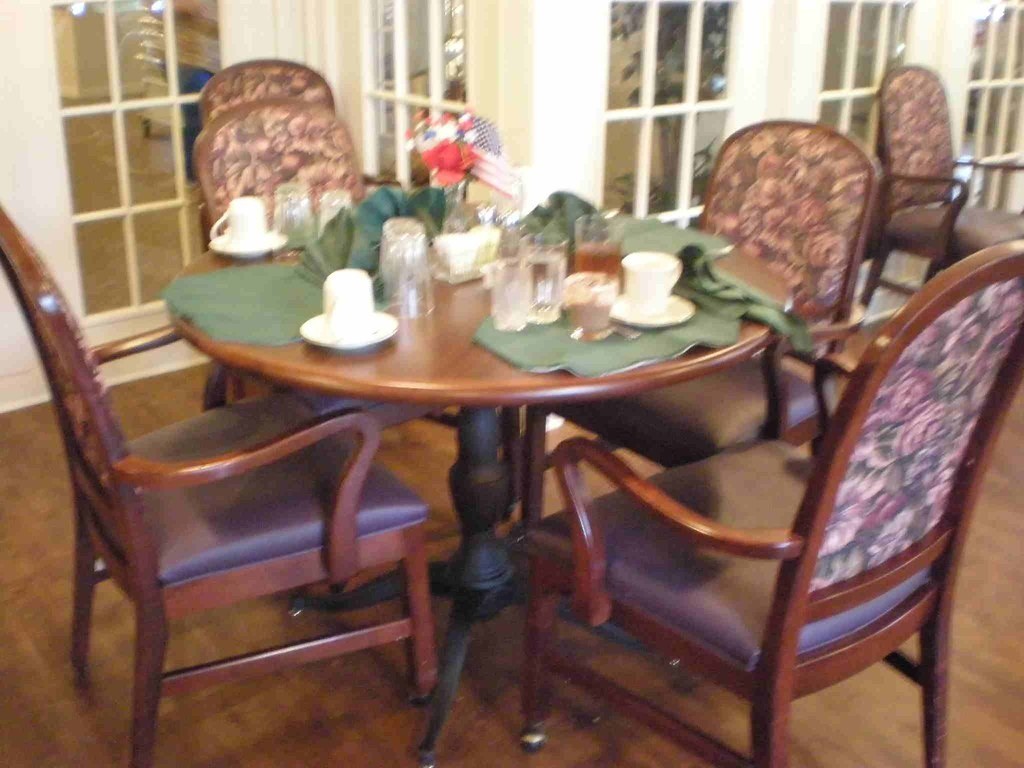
[291,204,358,282]
[474,299,742,381]
[520,190,610,254]
[348,182,448,313]
[670,243,815,354]
[605,213,733,264]
[159,262,346,348]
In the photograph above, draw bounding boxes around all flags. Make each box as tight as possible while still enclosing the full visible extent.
[468,117,514,199]
[413,114,477,172]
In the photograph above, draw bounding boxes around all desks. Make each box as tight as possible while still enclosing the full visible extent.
[168,248,795,768]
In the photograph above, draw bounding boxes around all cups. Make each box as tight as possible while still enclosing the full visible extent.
[565,271,617,332]
[380,218,435,319]
[572,215,626,274]
[620,250,683,315]
[274,183,314,246]
[210,197,269,249]
[434,187,522,283]
[322,268,374,342]
[319,189,353,235]
[520,232,567,323]
[488,260,526,333]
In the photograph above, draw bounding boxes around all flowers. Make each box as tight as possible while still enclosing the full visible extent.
[405,103,477,189]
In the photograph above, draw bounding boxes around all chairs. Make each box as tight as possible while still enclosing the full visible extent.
[857,63,1024,309]
[518,236,1024,768]
[521,120,879,531]
[0,208,438,768]
[190,98,452,440]
[196,59,402,186]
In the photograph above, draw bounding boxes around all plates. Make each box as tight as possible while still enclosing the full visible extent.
[610,294,697,329]
[209,235,288,258]
[300,313,398,349]
